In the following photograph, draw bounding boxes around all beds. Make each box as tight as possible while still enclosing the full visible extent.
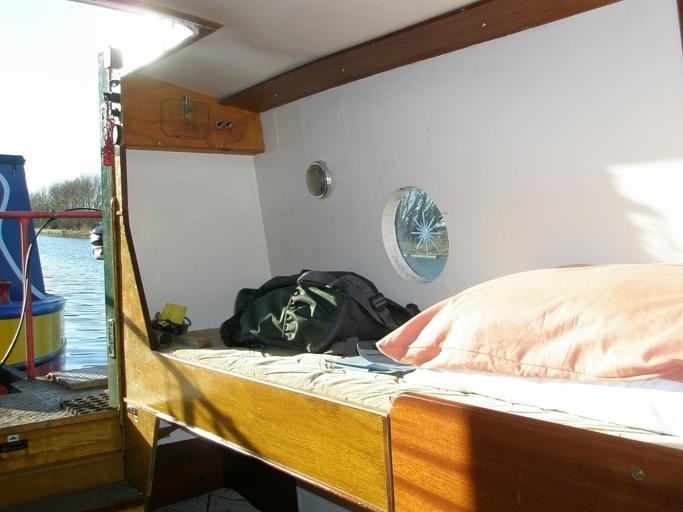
[109,70,683,509]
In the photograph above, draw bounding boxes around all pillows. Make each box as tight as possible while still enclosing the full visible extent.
[376,254,683,385]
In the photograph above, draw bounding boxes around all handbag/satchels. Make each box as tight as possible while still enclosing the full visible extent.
[220,269,418,352]
